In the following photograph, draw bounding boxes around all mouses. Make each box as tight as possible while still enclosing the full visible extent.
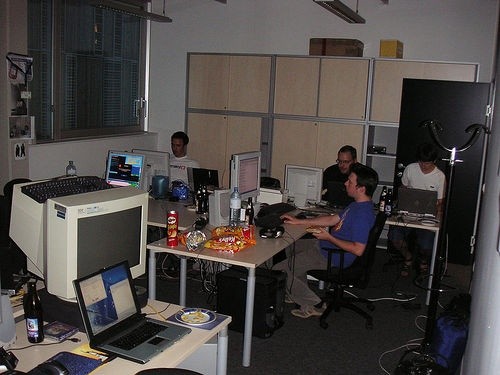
[38,359,69,375]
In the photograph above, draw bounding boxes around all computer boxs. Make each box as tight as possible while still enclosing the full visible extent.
[215,266,288,339]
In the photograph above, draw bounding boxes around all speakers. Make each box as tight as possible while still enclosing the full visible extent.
[208,189,231,226]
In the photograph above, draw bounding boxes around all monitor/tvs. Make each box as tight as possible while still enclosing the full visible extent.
[284,165,326,205]
[9,178,149,303]
[397,188,438,216]
[132,149,172,191]
[105,150,146,187]
[230,151,260,208]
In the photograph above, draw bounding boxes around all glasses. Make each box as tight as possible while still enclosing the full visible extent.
[336,159,355,164]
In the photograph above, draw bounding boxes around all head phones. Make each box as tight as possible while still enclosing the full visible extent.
[259,226,285,239]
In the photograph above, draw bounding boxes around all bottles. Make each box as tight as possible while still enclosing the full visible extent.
[245,197,254,226]
[229,187,241,227]
[194,184,208,214]
[24,281,44,344]
[379,186,387,212]
[66,160,76,176]
[384,189,393,217]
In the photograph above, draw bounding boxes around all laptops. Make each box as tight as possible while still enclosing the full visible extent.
[72,260,192,363]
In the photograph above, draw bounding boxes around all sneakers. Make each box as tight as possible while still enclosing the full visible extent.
[284,294,294,302]
[291,306,322,318]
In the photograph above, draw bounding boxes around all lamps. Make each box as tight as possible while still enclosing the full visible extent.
[88,0,173,24]
[313,0,366,24]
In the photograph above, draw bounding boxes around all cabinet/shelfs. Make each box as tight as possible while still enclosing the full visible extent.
[184,51,480,249]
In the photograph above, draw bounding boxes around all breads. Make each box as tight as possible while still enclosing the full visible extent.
[306,225,326,233]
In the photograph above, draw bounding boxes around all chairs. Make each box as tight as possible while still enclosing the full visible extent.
[307,212,388,328]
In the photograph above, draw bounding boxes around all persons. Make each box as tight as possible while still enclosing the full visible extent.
[322,145,367,207]
[387,149,446,276]
[168,131,200,190]
[272,166,378,319]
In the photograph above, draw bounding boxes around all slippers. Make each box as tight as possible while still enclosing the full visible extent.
[420,262,429,273]
[400,262,412,277]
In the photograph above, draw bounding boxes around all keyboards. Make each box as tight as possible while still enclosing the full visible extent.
[21,175,113,203]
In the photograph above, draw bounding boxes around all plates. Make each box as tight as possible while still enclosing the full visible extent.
[175,308,216,325]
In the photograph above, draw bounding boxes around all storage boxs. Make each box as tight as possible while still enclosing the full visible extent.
[379,39,404,59]
[308,36,365,58]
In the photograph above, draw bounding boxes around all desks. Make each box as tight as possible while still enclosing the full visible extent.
[0,187,443,375]
